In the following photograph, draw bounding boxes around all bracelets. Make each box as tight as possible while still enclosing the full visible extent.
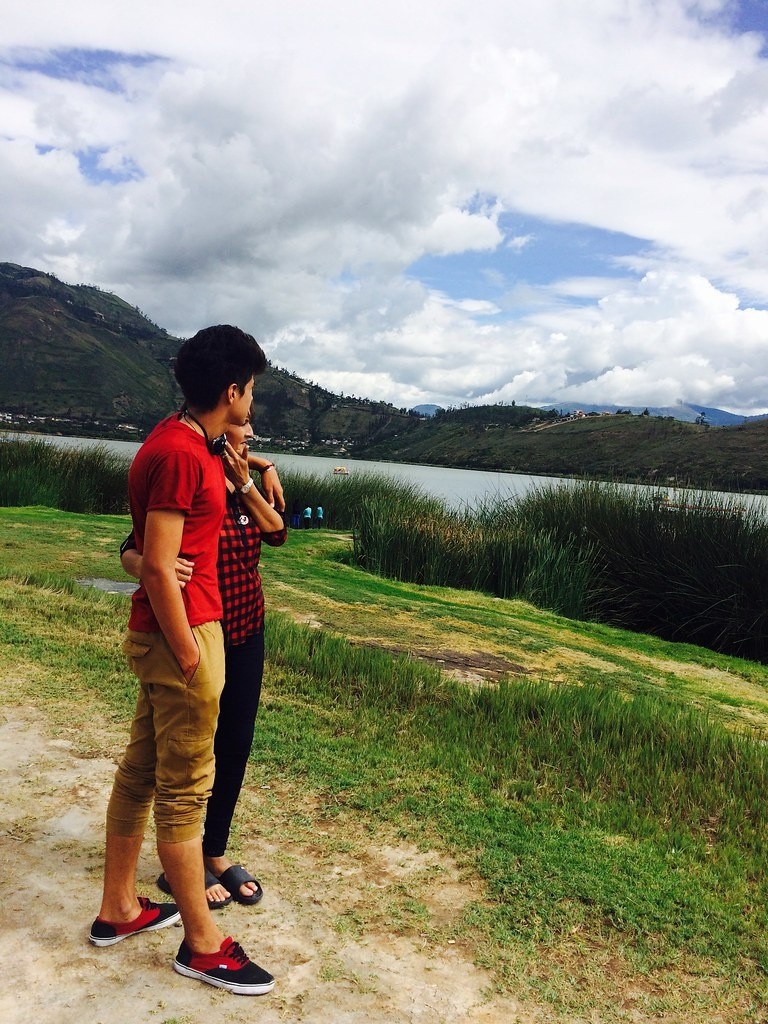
[261,463,274,473]
[238,479,253,494]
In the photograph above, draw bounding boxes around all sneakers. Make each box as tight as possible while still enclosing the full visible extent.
[173,936,276,996]
[89,897,181,948]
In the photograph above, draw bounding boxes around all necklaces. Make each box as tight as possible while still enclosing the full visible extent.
[184,415,196,431]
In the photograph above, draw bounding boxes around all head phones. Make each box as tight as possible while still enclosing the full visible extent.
[184,409,227,456]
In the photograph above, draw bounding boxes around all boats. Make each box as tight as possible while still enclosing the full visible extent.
[333,467,349,475]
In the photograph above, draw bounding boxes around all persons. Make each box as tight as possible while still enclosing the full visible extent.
[303,503,312,529]
[293,500,301,528]
[89,324,286,995]
[314,504,324,527]
[121,403,287,909]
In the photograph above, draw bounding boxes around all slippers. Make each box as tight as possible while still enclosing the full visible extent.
[157,868,233,908]
[220,865,263,904]
